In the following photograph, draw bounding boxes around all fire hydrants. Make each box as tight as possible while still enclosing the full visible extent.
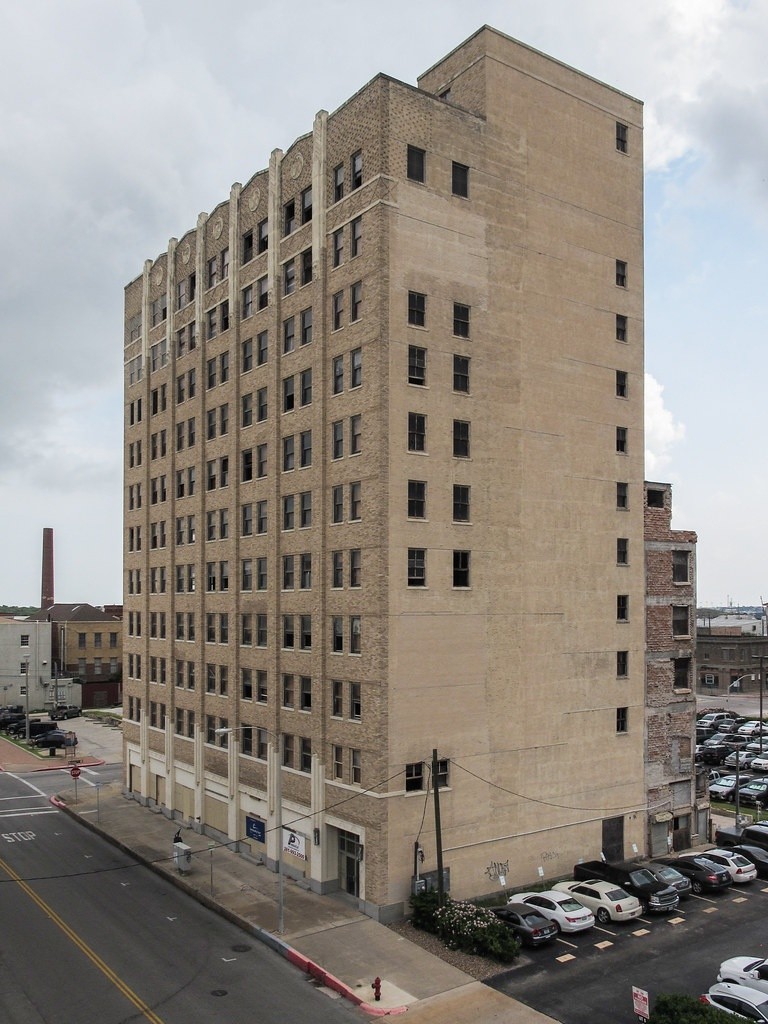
[372,976,381,1000]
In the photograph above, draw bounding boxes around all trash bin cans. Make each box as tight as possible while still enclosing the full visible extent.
[49,746,56,757]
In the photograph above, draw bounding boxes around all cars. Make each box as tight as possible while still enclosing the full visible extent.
[720,845,768,877]
[695,719,768,766]
[724,751,759,770]
[738,777,768,808]
[0,704,78,749]
[650,857,735,895]
[717,956,768,996]
[750,750,768,773]
[678,847,758,886]
[507,890,596,935]
[551,877,643,925]
[484,902,560,949]
[626,860,692,898]
[698,981,768,1024]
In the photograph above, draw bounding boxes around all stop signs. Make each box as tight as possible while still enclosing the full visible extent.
[70,766,82,779]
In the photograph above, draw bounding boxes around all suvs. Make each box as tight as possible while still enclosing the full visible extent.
[709,774,750,803]
[697,713,732,730]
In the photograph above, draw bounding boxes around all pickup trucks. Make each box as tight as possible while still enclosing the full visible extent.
[715,824,768,852]
[573,859,680,917]
[49,704,82,721]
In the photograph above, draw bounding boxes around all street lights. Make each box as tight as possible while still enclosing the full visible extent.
[214,726,285,933]
[23,653,32,743]
[751,656,768,752]
